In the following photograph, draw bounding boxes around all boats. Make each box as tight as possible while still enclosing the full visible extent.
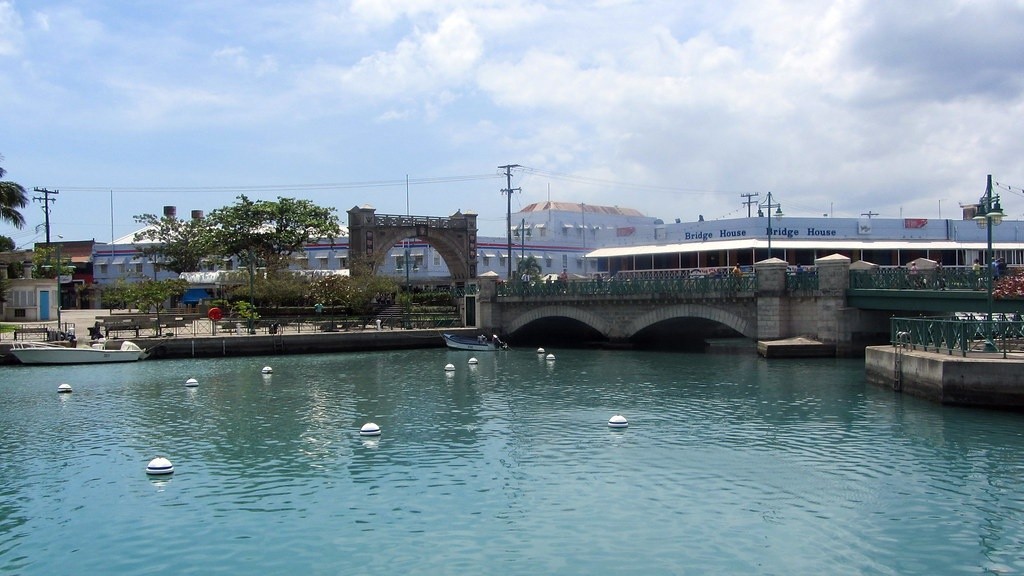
[10,340,151,365]
[441,332,514,352]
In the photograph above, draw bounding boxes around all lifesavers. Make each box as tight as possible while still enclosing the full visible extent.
[208,308,222,321]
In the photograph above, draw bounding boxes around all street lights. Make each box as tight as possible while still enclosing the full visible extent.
[972,195,1008,352]
[40,265,78,331]
[512,228,535,263]
[757,203,785,258]
